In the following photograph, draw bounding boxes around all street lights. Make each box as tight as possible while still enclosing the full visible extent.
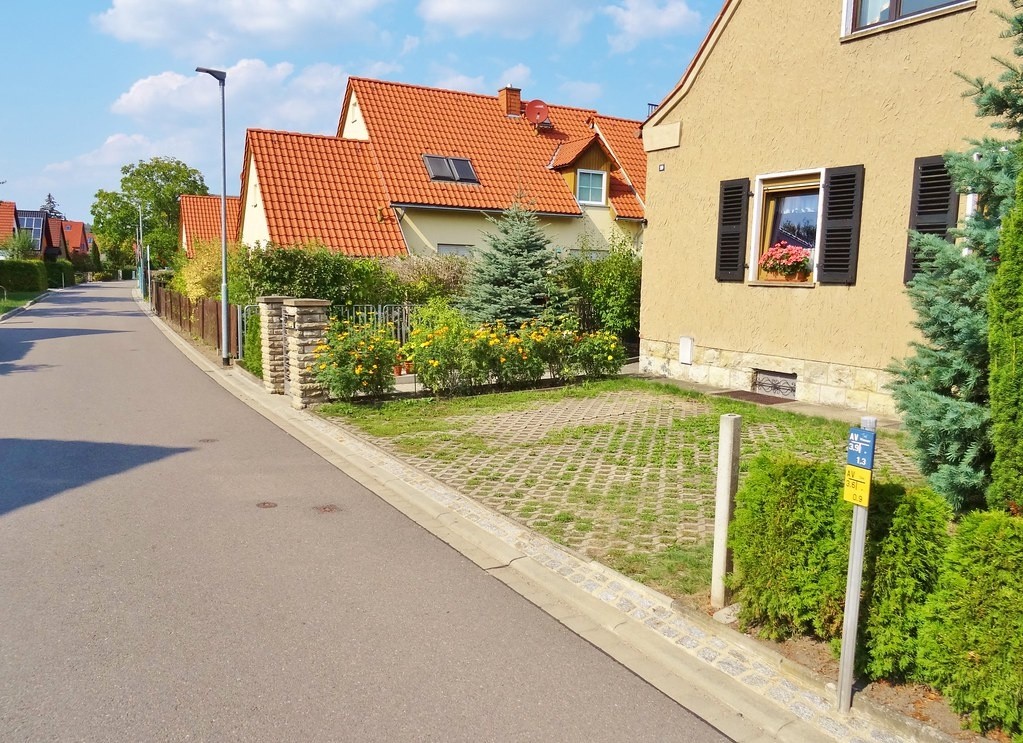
[132,201,142,262]
[195,66,232,367]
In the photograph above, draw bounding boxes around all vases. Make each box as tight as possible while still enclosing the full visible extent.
[404,362,413,373]
[393,366,401,375]
[765,267,806,281]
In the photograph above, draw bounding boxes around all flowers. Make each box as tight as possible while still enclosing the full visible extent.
[758,239,810,275]
